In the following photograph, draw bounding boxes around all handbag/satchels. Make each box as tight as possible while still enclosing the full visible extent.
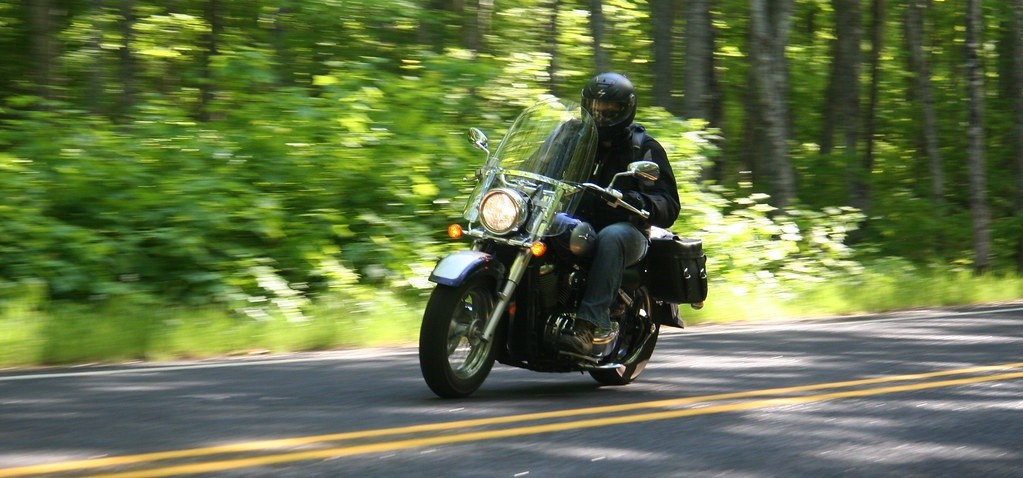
[646,238,709,303]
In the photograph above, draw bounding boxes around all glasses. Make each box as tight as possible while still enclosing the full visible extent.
[591,107,622,118]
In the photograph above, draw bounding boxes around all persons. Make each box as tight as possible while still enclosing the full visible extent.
[525,71,682,355]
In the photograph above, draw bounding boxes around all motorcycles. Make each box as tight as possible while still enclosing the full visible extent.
[417,97,706,400]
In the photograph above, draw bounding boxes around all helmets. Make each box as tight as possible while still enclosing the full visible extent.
[581,72,636,139]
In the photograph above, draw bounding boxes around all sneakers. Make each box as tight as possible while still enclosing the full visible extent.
[558,319,595,357]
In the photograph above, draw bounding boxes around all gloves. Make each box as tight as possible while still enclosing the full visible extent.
[624,189,646,212]
